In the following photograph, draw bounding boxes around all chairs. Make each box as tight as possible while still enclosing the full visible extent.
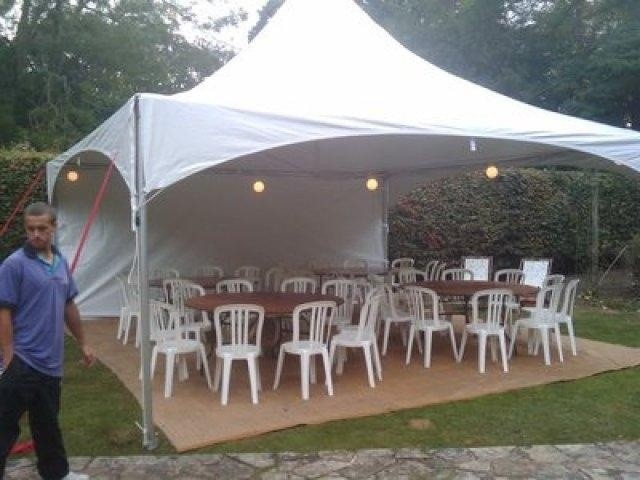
[116,255,551,356]
[456,290,512,375]
[325,295,387,388]
[402,285,459,370]
[523,273,566,342]
[535,279,580,357]
[213,303,265,406]
[271,301,336,401]
[508,285,563,366]
[149,299,211,396]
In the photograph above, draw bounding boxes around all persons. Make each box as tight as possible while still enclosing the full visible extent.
[2,202,95,479]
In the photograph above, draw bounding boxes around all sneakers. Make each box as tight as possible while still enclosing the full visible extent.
[62,472,89,480]
